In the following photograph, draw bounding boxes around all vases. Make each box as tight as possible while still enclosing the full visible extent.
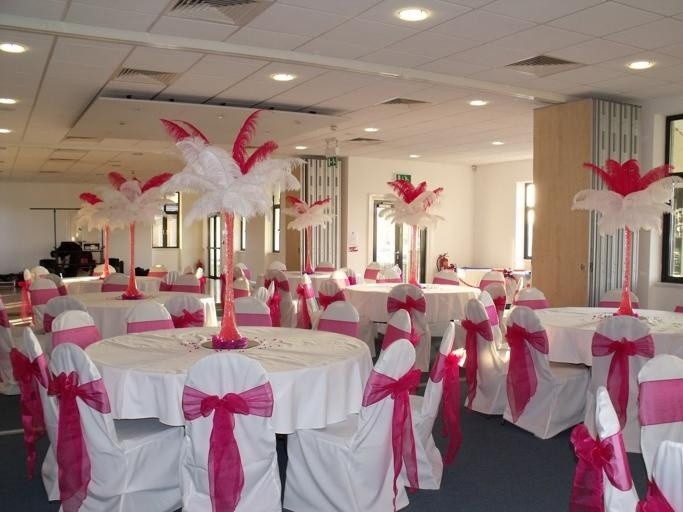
[616,225,635,317]
[217,211,243,342]
[302,224,315,276]
[407,223,421,289]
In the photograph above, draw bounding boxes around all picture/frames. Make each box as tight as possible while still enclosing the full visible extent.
[99,223,152,302]
